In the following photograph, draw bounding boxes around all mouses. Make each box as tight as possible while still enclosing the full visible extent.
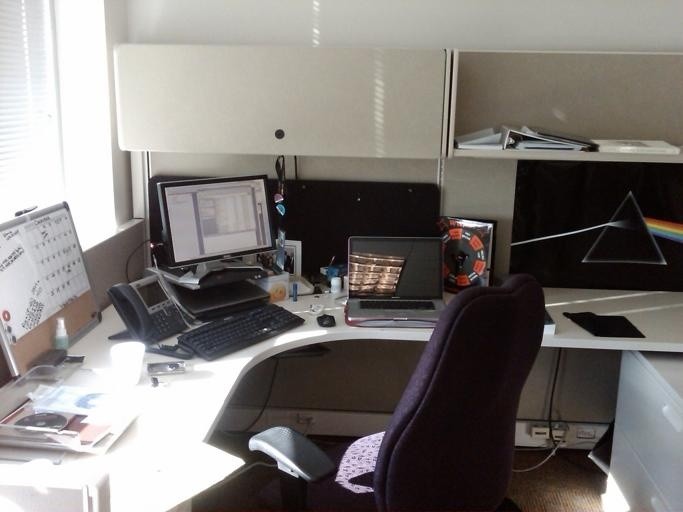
[317,313,336,327]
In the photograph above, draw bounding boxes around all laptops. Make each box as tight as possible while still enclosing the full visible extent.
[345,234,448,328]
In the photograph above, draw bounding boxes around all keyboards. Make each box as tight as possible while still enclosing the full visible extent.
[176,304,305,362]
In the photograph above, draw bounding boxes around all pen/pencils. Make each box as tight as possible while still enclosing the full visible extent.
[329,256,335,266]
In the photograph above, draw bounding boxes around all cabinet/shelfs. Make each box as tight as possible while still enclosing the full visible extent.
[113,41,683,164]
[598,350,683,511]
[246,273,545,512]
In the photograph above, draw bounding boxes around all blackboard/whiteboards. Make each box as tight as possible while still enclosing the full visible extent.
[0,202,103,386]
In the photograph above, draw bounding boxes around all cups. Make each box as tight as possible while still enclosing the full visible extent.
[111,340,145,385]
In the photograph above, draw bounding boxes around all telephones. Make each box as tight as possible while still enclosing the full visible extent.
[107,275,189,347]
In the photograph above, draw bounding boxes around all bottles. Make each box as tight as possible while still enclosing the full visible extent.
[330,278,341,293]
[55,316,68,351]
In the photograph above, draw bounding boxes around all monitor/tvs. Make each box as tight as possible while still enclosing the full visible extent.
[508,159,683,293]
[148,174,278,285]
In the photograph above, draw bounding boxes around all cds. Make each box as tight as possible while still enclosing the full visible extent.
[15,412,69,434]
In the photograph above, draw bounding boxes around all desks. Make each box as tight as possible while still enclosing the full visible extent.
[0,280,683,512]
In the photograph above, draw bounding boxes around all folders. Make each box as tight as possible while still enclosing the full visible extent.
[501,123,600,153]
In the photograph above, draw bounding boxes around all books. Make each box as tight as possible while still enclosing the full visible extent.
[591,139,680,155]
